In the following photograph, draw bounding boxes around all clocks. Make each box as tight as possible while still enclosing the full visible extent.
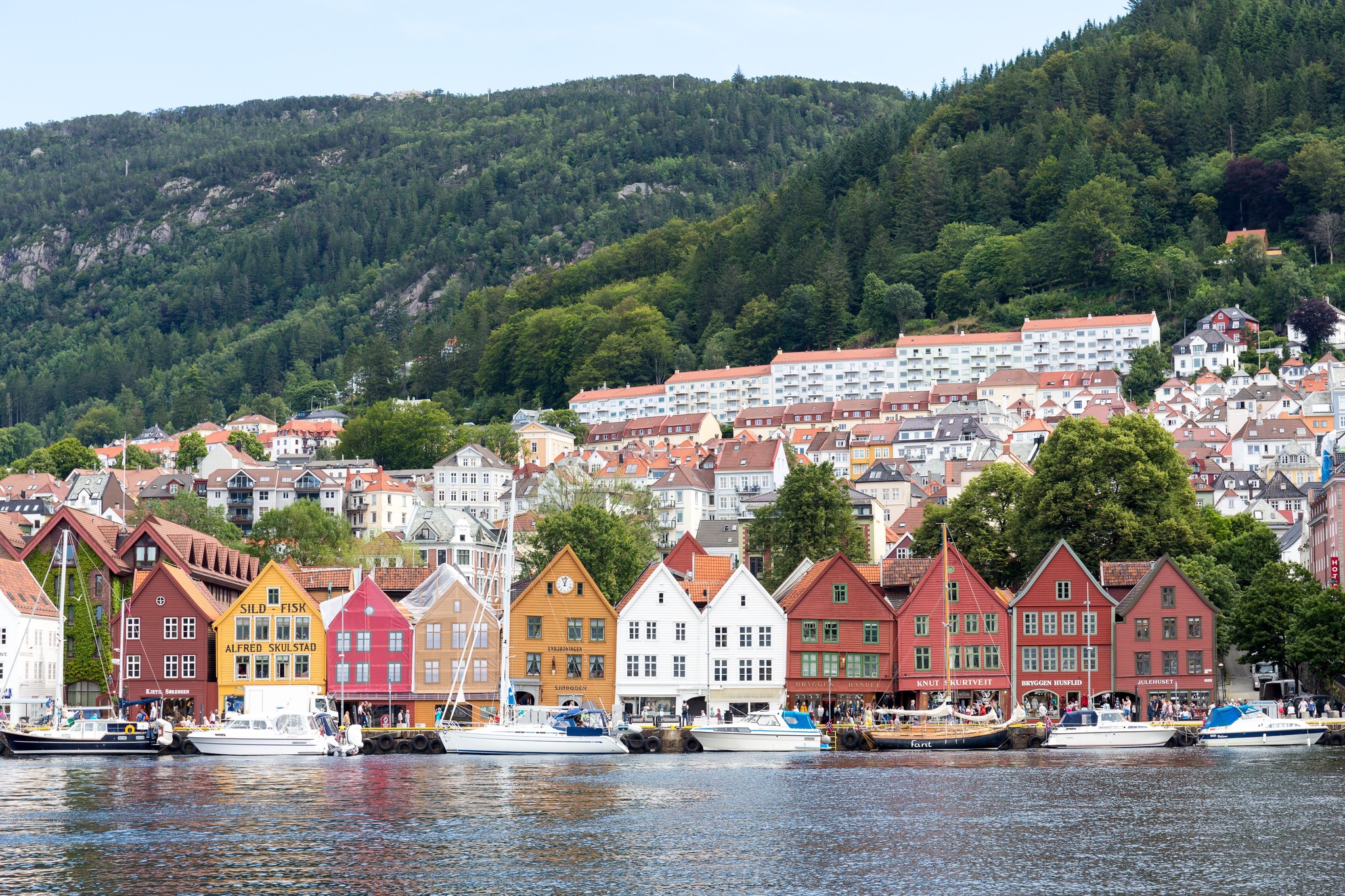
[555,574,575,595]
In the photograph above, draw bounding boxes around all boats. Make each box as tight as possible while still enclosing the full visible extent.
[1040,708,1178,748]
[184,705,337,757]
[1193,705,1330,748]
[687,708,825,754]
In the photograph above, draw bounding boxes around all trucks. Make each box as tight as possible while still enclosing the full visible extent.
[1251,661,1279,691]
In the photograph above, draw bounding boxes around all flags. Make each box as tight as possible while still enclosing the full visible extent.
[1046,717,1051,731]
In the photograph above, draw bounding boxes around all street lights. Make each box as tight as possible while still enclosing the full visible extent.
[1082,581,1093,711]
[1218,663,1226,702]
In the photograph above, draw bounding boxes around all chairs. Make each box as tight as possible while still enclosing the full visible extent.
[743,486,748,492]
[750,486,757,492]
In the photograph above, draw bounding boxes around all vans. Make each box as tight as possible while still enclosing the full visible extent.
[1259,679,1309,704]
[1283,693,1345,718]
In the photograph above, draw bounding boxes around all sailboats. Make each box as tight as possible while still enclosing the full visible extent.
[857,520,1028,751]
[0,525,177,755]
[432,477,631,757]
[327,588,359,756]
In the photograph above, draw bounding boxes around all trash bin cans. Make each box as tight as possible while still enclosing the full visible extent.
[21,716,30,727]
[656,716,662,727]
[66,718,73,727]
[724,710,733,724]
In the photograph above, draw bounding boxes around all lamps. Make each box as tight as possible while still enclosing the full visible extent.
[1330,578,1337,583]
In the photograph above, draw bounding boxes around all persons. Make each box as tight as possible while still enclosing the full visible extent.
[435,707,443,727]
[959,696,1345,725]
[568,701,597,727]
[343,711,351,729]
[398,710,403,723]
[620,701,665,721]
[488,711,495,725]
[1031,698,1038,709]
[701,709,720,720]
[0,698,227,728]
[793,704,921,731]
[495,710,500,725]
[681,701,689,727]
[226,694,235,711]
[795,700,861,720]
[358,701,372,728]
[405,710,410,728]
[905,700,916,724]
[883,693,895,716]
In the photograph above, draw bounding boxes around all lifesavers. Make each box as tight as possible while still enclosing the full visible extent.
[1165,735,1178,748]
[859,738,871,751]
[396,740,414,754]
[125,725,135,734]
[1316,731,1328,744]
[359,738,376,755]
[429,739,445,754]
[379,734,394,751]
[0,740,4,753]
[1329,733,1344,748]
[167,733,182,750]
[1028,735,1045,748]
[1178,731,1195,746]
[181,740,196,755]
[645,737,661,752]
[629,733,644,749]
[999,736,1013,750]
[842,730,859,749]
[684,737,701,752]
[620,737,628,749]
[411,734,428,751]
